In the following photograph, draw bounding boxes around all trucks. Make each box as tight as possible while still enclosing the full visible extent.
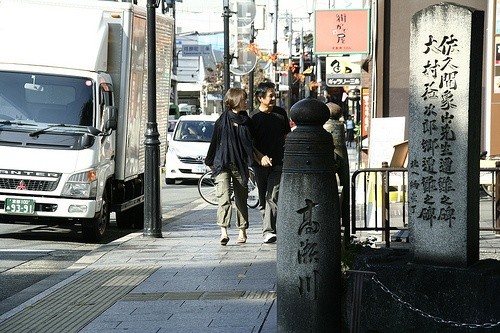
[0,0,174,241]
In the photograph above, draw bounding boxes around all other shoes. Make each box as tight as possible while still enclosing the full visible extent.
[220,236,229,246]
[236,235,247,243]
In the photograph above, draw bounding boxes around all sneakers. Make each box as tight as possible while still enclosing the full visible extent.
[263,233,276,243]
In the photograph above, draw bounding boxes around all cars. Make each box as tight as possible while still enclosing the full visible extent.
[165,115,225,185]
[168,103,195,131]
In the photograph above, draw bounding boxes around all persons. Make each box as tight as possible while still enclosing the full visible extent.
[248,81,292,243]
[345,115,355,146]
[204,88,272,245]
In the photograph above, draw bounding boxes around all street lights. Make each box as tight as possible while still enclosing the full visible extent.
[131,0,180,238]
[248,1,265,118]
[221,0,240,88]
[268,1,312,109]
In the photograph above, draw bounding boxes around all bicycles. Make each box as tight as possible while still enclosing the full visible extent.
[198,166,261,209]
